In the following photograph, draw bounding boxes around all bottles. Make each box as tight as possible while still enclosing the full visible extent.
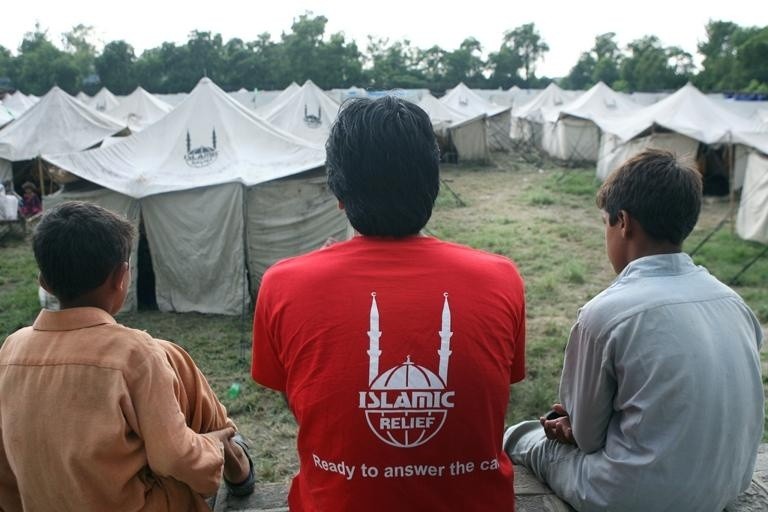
[230,383,240,398]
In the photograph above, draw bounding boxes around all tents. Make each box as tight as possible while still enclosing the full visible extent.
[0,77,768,314]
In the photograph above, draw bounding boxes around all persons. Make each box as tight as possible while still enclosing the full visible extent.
[502,148,765,512]
[251,95,525,512]
[1,202,255,512]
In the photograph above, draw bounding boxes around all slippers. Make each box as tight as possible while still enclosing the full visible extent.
[223,432,255,498]
